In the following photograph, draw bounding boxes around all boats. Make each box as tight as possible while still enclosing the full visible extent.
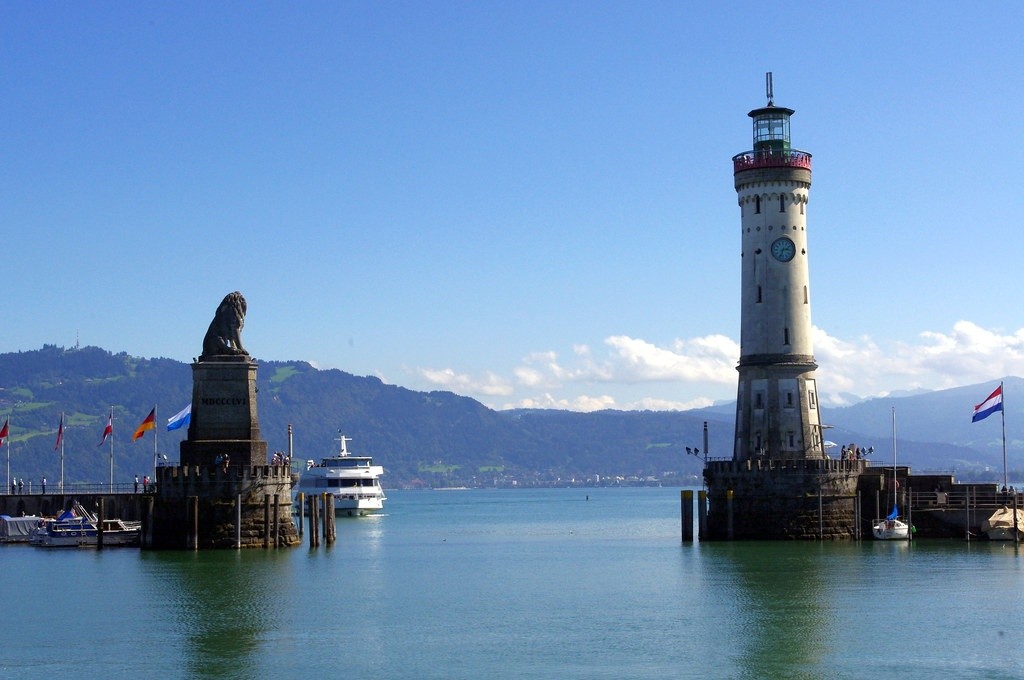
[30,512,140,548]
[0,515,43,542]
[293,435,389,518]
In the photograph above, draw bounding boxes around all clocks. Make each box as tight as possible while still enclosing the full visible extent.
[771,237,796,262]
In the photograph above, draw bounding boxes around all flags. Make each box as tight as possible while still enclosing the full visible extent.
[55,420,62,450]
[167,404,191,431]
[132,408,154,444]
[96,415,112,447]
[0,421,8,447]
[972,386,1003,422]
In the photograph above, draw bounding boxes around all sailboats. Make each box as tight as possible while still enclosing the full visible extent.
[871,404,910,540]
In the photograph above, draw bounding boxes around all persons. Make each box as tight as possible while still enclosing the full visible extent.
[143,476,147,492]
[841,445,861,470]
[18,478,24,494]
[741,153,751,166]
[133,475,138,493]
[215,453,230,473]
[42,477,46,494]
[11,478,16,495]
[271,450,289,474]
[1001,485,1014,504]
